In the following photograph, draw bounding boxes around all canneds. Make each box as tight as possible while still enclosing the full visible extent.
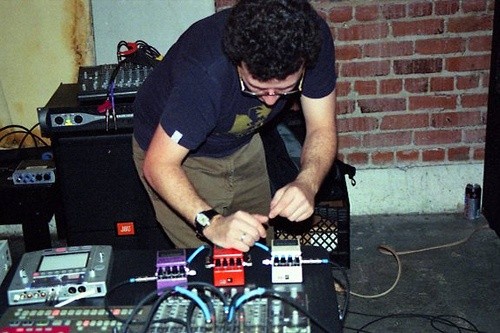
[464,184,481,220]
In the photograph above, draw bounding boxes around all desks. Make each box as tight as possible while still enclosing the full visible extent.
[0,146,58,255]
[0,244,340,332]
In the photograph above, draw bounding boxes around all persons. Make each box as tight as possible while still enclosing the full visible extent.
[132,1,338,250]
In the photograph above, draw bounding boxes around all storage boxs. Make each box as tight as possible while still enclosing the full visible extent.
[273,178,351,269]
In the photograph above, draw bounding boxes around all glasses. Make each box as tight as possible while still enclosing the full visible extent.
[239,66,304,95]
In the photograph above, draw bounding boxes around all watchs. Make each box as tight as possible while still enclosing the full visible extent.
[193,208,218,235]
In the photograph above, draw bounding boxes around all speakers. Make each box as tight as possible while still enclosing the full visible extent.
[52,133,175,252]
